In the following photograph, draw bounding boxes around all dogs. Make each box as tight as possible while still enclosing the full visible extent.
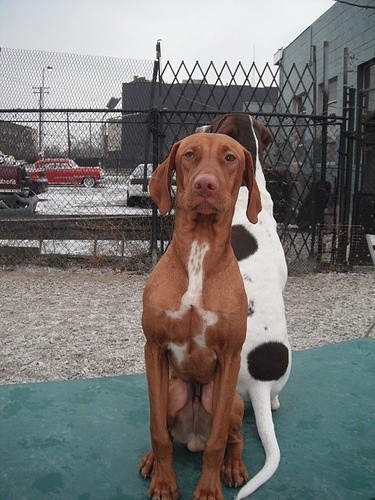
[137,132,262,500]
[195,115,291,500]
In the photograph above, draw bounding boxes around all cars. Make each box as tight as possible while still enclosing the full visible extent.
[28,158,104,188]
[126,162,179,209]
